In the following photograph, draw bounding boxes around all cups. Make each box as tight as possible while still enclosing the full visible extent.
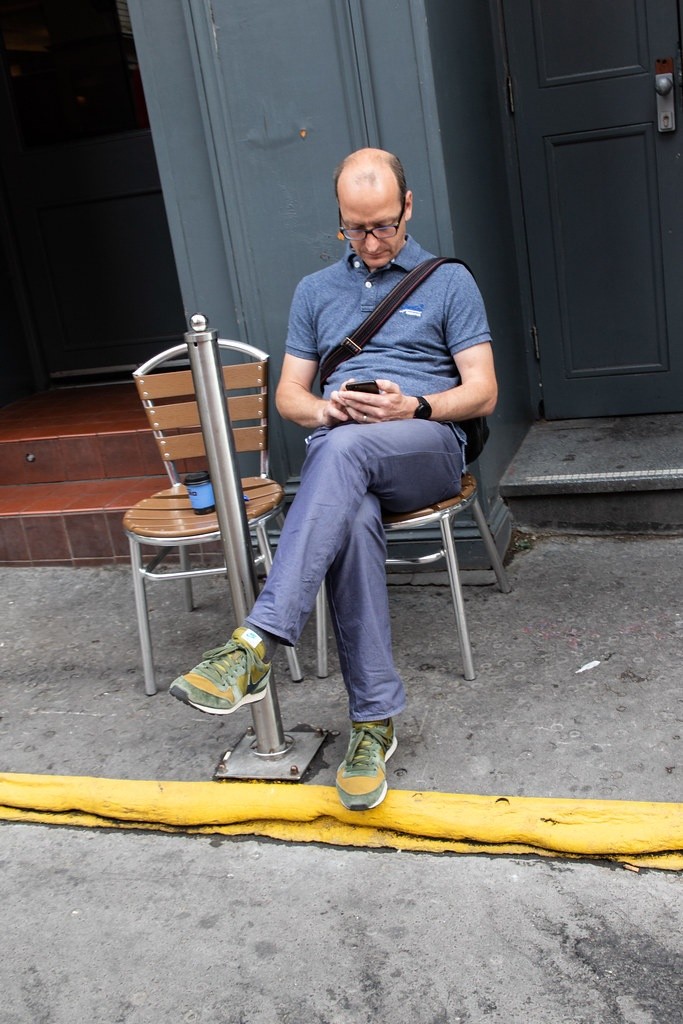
[182,471,216,515]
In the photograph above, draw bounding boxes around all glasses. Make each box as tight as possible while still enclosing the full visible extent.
[338,196,405,240]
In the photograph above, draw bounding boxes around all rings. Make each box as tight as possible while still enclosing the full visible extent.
[361,414,368,423]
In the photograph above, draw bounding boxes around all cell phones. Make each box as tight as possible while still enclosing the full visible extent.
[346,380,380,395]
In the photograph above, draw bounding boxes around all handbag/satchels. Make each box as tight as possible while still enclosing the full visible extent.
[458,417,490,464]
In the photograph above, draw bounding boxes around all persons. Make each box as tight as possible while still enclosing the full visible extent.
[170,146,498,811]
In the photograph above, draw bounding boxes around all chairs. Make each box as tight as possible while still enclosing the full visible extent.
[121,336,303,694]
[314,467,512,679]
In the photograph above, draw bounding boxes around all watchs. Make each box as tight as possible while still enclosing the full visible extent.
[412,394,433,421]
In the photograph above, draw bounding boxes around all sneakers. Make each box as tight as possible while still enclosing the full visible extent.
[169,628,271,716]
[335,717,398,811]
